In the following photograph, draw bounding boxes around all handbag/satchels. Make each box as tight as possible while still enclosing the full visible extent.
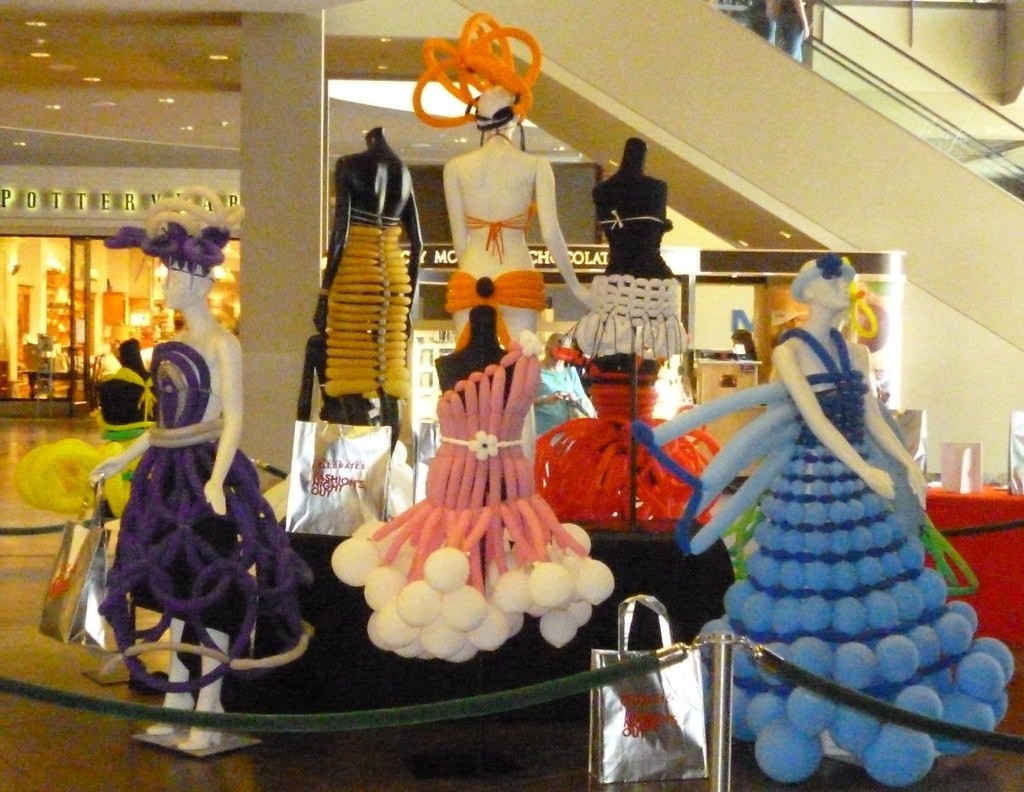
[35,475,110,648]
[285,417,393,538]
[586,595,709,784]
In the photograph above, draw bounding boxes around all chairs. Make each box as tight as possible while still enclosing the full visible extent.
[68,354,105,414]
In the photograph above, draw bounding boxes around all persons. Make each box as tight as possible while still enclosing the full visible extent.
[716,0,809,62]
[89,186,316,748]
[262,128,422,524]
[15,337,158,517]
[731,330,758,361]
[533,138,721,532]
[331,306,615,663]
[414,13,599,467]
[632,255,1014,785]
[535,333,597,438]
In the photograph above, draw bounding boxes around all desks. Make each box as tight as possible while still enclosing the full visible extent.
[23,371,92,398]
[924,487,1024,654]
